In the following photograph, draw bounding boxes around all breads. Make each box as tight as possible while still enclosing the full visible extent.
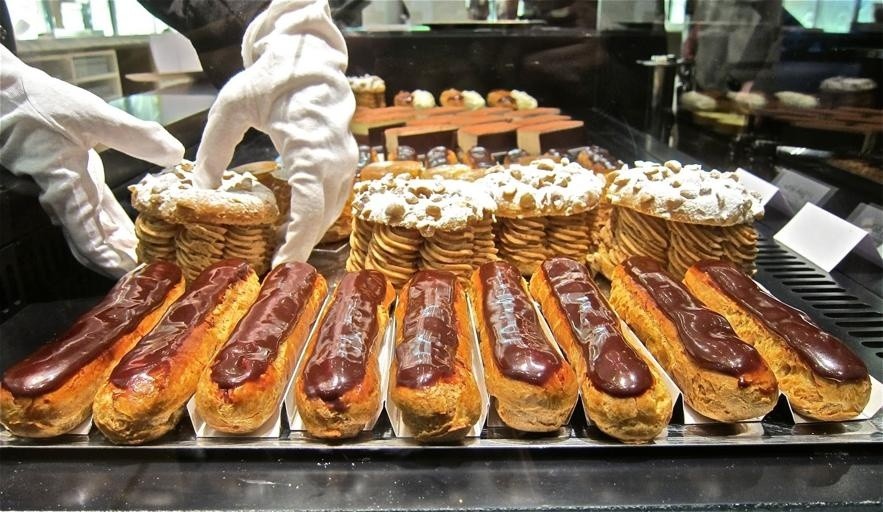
[393,88,436,107]
[128,144,764,295]
[439,88,486,108]
[488,90,539,110]
[345,72,388,107]
[1,258,871,445]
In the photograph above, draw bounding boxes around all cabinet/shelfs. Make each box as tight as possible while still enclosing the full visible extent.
[22,49,123,103]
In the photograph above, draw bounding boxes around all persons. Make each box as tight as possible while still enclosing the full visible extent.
[0,0,359,282]
[471,2,783,95]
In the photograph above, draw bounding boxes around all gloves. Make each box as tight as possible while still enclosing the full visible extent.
[1,43,187,279]
[191,0,360,271]
